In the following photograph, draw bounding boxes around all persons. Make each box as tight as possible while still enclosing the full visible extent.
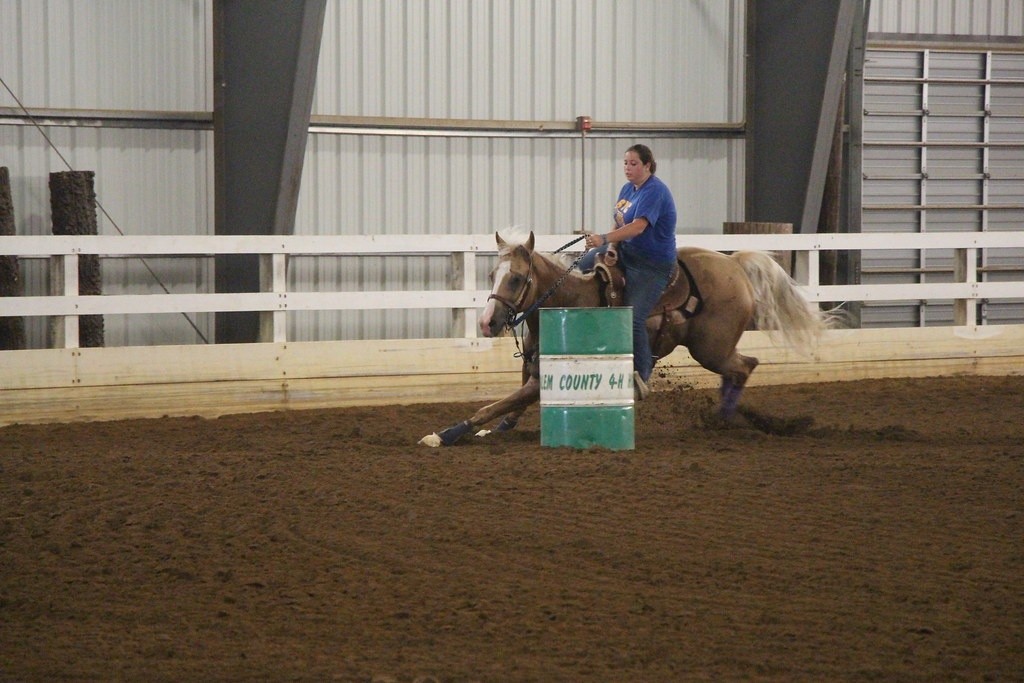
[577,143,679,388]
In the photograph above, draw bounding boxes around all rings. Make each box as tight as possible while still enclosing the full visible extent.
[589,240,591,243]
[591,242,594,246]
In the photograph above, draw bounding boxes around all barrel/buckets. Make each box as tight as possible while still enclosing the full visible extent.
[721,220,794,277]
[537,306,635,451]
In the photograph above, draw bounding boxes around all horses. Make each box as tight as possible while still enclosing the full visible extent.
[415,231,859,449]
